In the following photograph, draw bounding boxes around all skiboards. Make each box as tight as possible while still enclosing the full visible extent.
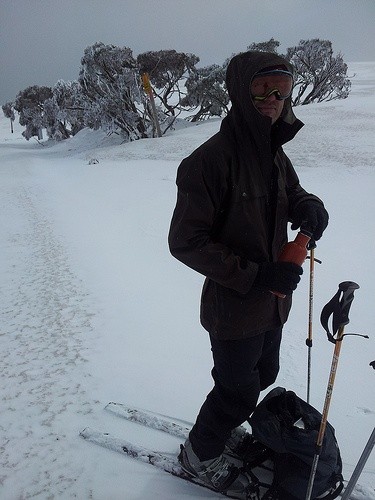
[80,402,375,500]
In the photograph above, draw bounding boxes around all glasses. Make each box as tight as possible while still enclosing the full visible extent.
[250,70,294,100]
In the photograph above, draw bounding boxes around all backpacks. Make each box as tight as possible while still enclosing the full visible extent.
[241,387,344,500]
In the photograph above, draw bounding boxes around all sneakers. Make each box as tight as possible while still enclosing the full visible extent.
[177,437,250,498]
[224,426,255,461]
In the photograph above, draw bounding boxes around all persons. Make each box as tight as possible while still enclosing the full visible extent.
[167,50,329,499]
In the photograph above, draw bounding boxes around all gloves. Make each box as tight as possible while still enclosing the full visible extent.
[291,200,329,240]
[251,261,303,296]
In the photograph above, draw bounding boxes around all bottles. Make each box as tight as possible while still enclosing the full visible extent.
[268,230,312,298]
